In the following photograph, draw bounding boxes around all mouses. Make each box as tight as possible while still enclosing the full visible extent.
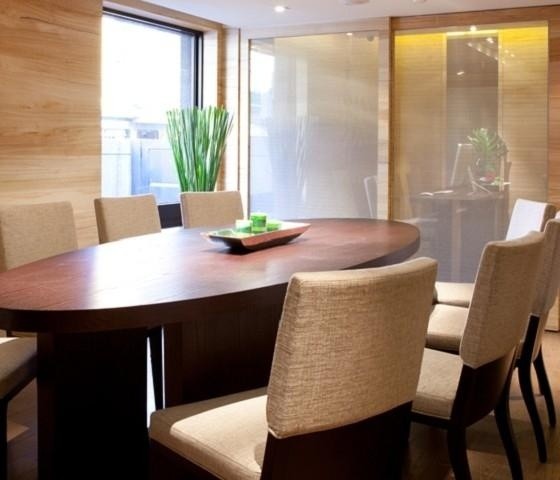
[420,192,433,196]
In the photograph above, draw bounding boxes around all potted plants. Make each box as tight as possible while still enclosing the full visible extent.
[468,127,509,182]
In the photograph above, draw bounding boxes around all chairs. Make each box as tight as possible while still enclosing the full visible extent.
[0,337,44,479]
[430,198,553,397]
[426,217,559,463]
[0,199,79,338]
[408,229,550,480]
[180,190,244,229]
[148,256,441,479]
[92,193,163,244]
[365,172,444,263]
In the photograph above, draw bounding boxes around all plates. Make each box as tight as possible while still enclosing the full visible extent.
[200,220,312,252]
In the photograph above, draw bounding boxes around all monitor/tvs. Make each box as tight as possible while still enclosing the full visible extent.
[450,143,477,196]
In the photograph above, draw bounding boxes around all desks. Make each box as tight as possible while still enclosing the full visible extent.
[423,186,506,287]
[0,218,421,479]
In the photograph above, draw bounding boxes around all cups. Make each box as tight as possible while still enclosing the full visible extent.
[232,211,281,235]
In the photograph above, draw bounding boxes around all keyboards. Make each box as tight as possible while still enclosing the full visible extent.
[434,190,452,193]
